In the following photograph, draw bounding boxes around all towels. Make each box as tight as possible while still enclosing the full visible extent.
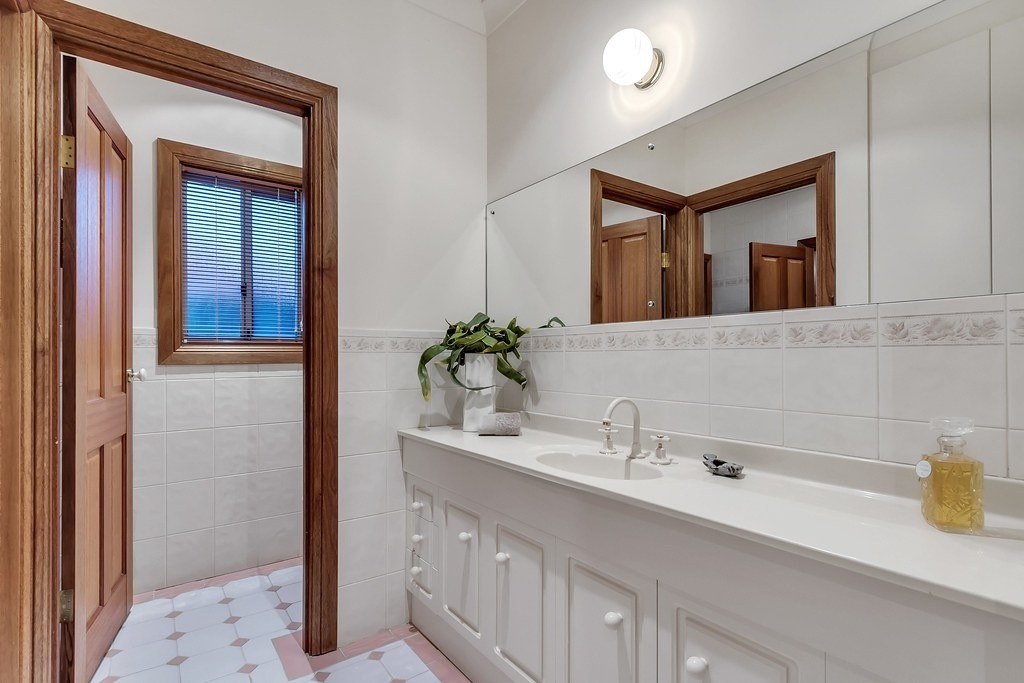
[478,413,521,436]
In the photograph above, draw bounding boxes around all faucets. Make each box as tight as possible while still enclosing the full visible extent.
[602,397,640,443]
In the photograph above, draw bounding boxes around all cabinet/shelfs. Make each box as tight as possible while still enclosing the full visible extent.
[400,424,1024,683]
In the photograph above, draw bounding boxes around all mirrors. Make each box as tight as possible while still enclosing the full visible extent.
[484,0,1024,332]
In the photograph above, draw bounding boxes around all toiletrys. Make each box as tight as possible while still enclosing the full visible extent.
[914,417,983,536]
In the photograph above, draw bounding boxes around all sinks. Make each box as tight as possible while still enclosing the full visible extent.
[535,453,664,481]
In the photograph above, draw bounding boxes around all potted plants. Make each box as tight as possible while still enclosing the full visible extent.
[417,314,529,434]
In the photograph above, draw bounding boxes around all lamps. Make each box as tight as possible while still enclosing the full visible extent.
[603,28,665,90]
